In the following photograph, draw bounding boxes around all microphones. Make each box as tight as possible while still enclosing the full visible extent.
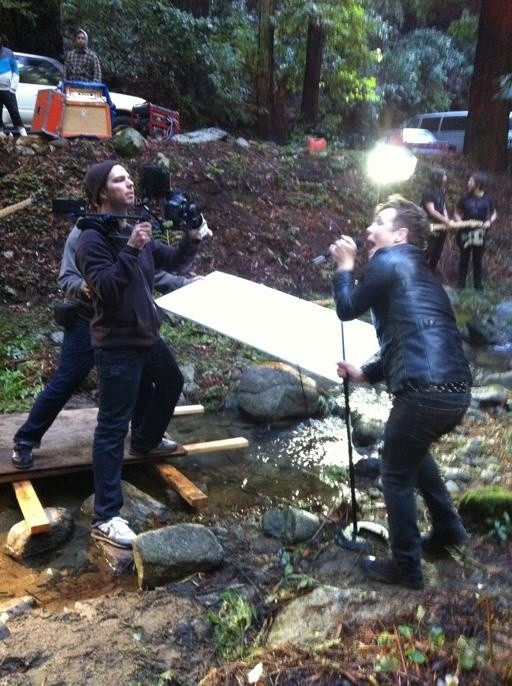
[310,237,364,266]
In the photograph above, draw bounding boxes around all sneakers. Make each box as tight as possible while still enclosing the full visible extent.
[127,436,178,457]
[18,127,28,136]
[92,515,138,550]
[11,443,34,469]
[0,131,7,137]
[420,526,471,553]
[357,554,426,590]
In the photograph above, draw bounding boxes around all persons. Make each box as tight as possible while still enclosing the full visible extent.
[74,161,213,549]
[0,35,28,137]
[453,170,496,291]
[328,193,473,591]
[11,218,179,469]
[62,29,102,84]
[421,166,451,273]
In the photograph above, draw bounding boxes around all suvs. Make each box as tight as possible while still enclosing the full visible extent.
[2,47,152,141]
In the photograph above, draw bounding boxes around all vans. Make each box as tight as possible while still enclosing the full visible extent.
[399,108,512,150]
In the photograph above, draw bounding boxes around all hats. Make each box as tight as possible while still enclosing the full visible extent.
[80,158,122,209]
